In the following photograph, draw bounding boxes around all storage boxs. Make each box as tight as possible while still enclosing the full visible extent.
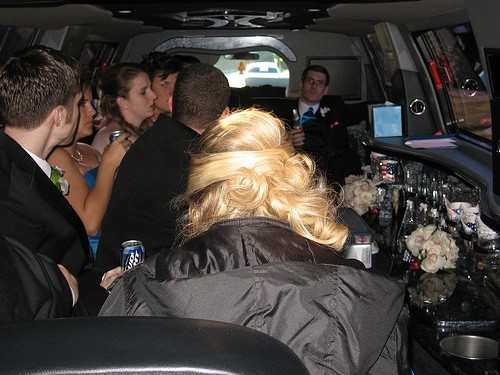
[367,103,402,138]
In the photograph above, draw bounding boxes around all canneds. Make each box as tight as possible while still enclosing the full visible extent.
[109,131,128,144]
[120,240,145,272]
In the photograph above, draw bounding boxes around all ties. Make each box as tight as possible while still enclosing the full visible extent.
[301,106,316,127]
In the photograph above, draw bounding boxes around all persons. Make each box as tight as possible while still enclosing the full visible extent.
[96,63,230,269]
[97,106,411,375]
[0,45,200,320]
[275,65,349,174]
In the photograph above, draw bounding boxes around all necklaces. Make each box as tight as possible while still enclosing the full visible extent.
[71,150,88,166]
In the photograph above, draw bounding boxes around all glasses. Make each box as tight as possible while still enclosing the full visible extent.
[306,79,325,86]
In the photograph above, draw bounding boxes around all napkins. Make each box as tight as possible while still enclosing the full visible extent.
[444,197,499,240]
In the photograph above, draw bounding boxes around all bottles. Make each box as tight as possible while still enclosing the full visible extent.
[292,109,300,130]
[390,169,482,301]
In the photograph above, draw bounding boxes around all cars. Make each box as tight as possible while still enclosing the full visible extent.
[238,62,281,75]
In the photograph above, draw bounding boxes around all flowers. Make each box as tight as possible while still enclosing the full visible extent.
[404,222,461,273]
[408,268,455,308]
[341,174,385,214]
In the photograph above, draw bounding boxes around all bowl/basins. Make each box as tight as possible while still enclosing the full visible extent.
[439,335,500,375]
